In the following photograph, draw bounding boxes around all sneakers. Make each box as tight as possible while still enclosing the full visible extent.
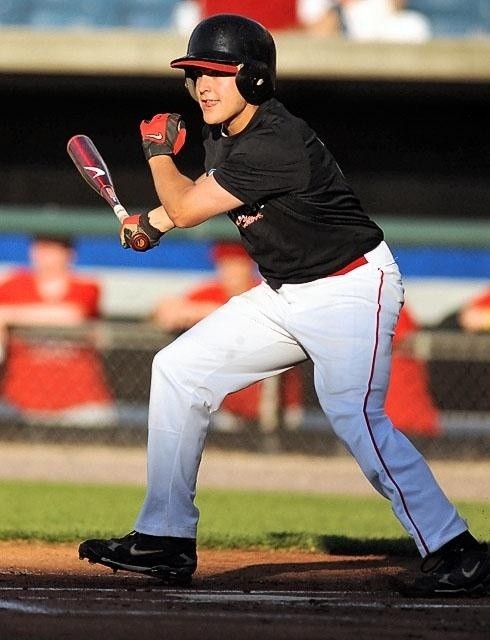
[386,540,490,600]
[76,528,198,580]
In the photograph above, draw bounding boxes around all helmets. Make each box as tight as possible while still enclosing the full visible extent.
[169,13,278,106]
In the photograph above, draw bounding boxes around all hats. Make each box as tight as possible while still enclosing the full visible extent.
[210,239,254,261]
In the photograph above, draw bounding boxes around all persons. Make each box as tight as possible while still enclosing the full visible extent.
[157,243,306,432]
[78,14,490,598]
[428,290,490,410]
[381,309,440,437]
[0,233,117,427]
[175,1,339,41]
[338,1,433,41]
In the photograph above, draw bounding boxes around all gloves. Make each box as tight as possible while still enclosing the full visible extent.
[140,111,187,161]
[119,211,164,252]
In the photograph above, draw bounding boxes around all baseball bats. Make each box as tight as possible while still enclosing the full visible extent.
[67,135,149,251]
